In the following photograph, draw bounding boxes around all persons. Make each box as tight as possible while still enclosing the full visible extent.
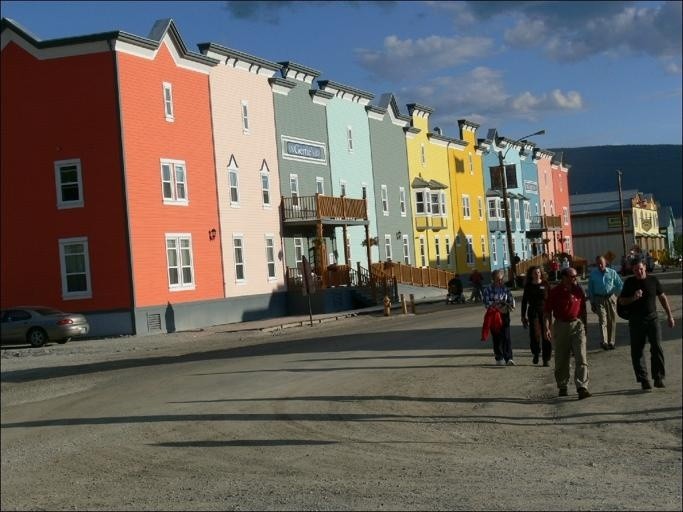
[447,254,674,399]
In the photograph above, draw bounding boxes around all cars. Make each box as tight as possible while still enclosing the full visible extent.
[0,303,92,349]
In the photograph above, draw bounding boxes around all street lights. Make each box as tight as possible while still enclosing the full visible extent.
[497,126,545,290]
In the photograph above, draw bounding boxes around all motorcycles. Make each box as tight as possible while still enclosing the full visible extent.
[473,287,481,302]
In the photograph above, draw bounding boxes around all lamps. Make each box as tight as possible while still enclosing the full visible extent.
[395,230,400,240]
[208,227,216,240]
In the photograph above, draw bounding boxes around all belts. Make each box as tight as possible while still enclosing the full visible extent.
[595,292,614,298]
[560,319,578,323]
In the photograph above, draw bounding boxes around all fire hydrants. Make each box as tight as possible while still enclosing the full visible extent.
[381,294,392,316]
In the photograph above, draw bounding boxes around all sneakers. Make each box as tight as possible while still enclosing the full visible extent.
[600,341,609,350]
[496,358,505,366]
[577,387,592,400]
[640,379,652,391]
[533,354,540,365]
[609,341,616,350]
[542,359,552,367]
[558,384,569,397]
[653,379,665,389]
[506,359,516,366]
